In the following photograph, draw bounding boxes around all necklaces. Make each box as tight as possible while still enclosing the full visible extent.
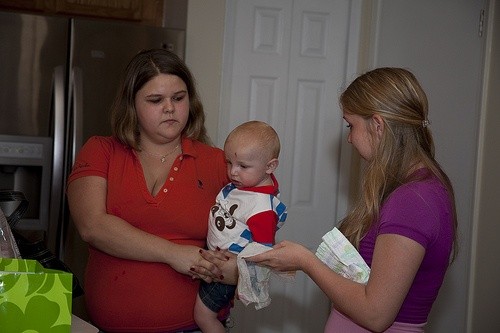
[151,144,178,163]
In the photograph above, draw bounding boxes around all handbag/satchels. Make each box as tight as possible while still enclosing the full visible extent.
[0,190,84,301]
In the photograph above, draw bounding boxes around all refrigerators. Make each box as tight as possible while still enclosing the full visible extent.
[0,6,188,291]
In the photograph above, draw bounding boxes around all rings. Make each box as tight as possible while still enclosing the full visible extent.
[204,270,208,275]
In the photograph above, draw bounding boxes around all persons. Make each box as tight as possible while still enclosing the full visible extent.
[66,49,238,333]
[194,121,287,333]
[245,67,459,333]
